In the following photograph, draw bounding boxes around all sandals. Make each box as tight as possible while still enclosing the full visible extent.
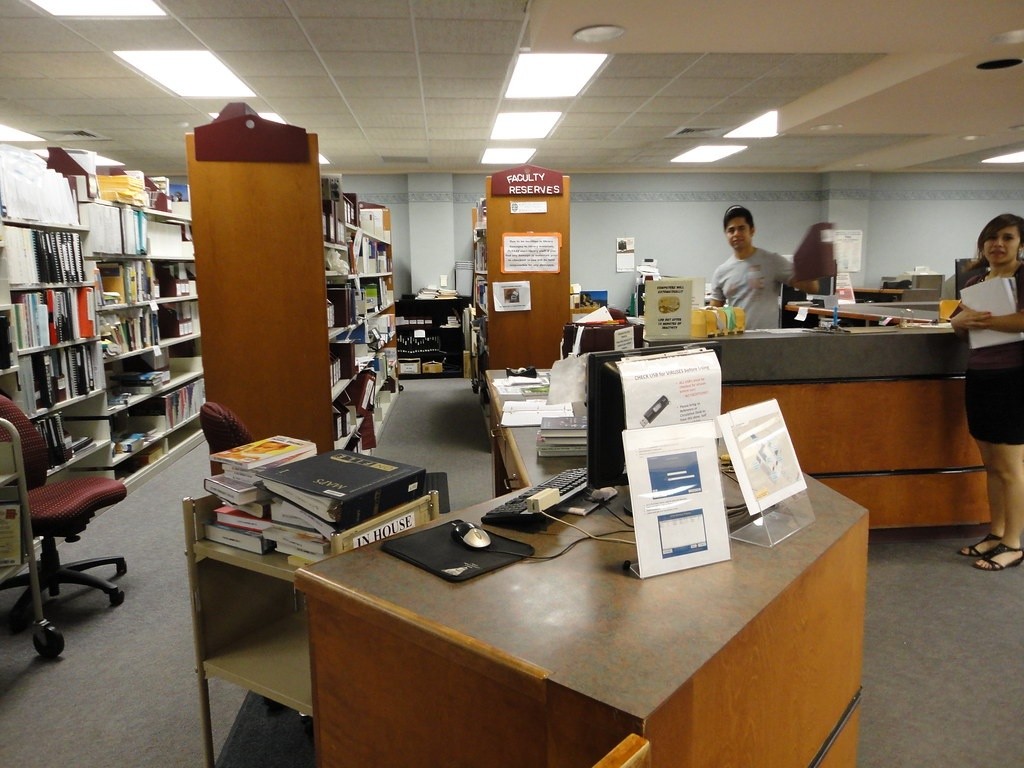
[958,533,1003,557]
[975,543,1024,571]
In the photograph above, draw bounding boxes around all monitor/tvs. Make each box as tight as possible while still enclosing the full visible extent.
[955,257,980,299]
[586,341,722,516]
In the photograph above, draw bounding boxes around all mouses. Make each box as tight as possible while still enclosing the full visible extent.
[451,522,492,551]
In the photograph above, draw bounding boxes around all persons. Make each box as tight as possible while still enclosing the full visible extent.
[707,204,836,331]
[950,214,1024,571]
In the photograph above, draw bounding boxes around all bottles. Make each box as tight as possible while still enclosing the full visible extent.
[640,395,669,426]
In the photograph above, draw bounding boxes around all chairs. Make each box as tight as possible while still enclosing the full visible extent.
[0,395,129,660]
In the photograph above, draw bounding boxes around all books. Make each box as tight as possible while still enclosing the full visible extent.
[536,416,587,456]
[203,434,426,568]
[961,277,1024,349]
[322,174,397,388]
[415,288,457,299]
[476,198,488,309]
[1,157,205,469]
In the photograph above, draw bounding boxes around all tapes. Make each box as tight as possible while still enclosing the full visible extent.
[518,367,526,373]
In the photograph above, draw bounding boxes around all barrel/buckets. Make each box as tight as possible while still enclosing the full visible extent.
[455,260,474,297]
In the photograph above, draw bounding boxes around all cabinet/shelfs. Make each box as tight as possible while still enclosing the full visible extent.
[0,101,573,659]
[180,489,439,768]
[853,288,937,302]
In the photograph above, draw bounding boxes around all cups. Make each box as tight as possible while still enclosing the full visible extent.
[900,309,914,328]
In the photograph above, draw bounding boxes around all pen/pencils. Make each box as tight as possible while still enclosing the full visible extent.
[834,306,838,326]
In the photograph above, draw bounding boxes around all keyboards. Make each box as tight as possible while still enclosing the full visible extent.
[481,467,587,524]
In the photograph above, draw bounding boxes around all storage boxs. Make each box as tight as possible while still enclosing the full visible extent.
[422,360,443,373]
[397,358,421,374]
[399,363,419,373]
